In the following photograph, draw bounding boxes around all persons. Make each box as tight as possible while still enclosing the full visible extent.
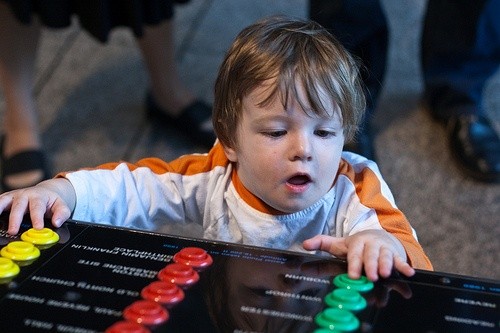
[0,0,219,191]
[200,244,414,333]
[307,0,500,183]
[0,12,441,278]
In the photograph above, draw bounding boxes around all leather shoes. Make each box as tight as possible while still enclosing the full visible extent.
[344,118,376,166]
[420,88,500,185]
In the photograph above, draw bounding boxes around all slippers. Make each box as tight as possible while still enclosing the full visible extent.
[0,130,53,191]
[143,89,217,146]
